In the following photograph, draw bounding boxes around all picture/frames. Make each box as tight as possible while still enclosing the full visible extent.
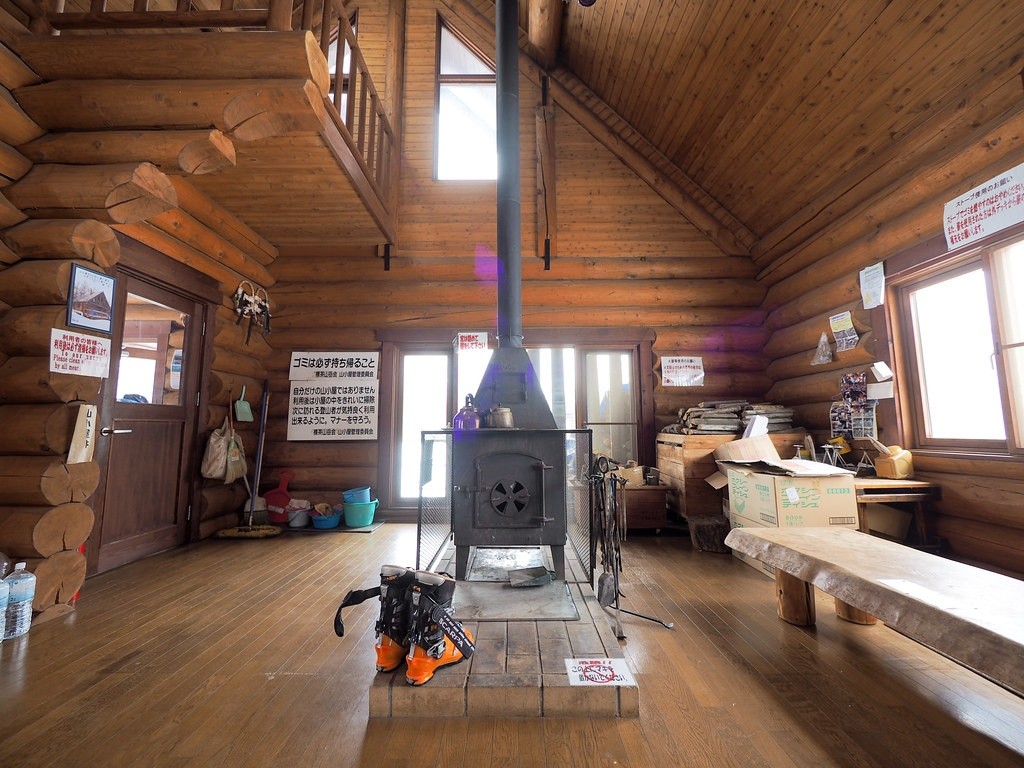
[65,261,117,336]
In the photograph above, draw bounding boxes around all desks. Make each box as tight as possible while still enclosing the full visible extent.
[852,475,950,557]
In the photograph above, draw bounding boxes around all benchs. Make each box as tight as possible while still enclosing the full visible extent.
[724,525,1024,700]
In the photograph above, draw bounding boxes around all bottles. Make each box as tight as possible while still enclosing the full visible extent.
[3,561,37,639]
[0,579,9,643]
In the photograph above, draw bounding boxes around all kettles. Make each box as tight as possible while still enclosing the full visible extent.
[452,393,484,430]
[484,403,514,428]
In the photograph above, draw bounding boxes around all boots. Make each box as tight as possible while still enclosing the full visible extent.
[375,565,476,685]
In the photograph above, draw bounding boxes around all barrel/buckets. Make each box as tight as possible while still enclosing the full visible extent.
[341,486,372,503]
[287,509,311,528]
[342,498,379,527]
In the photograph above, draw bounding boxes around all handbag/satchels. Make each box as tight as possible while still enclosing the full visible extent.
[201,416,245,479]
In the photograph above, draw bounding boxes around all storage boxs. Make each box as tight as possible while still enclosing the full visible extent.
[656,430,812,551]
[704,433,860,581]
[568,472,672,545]
